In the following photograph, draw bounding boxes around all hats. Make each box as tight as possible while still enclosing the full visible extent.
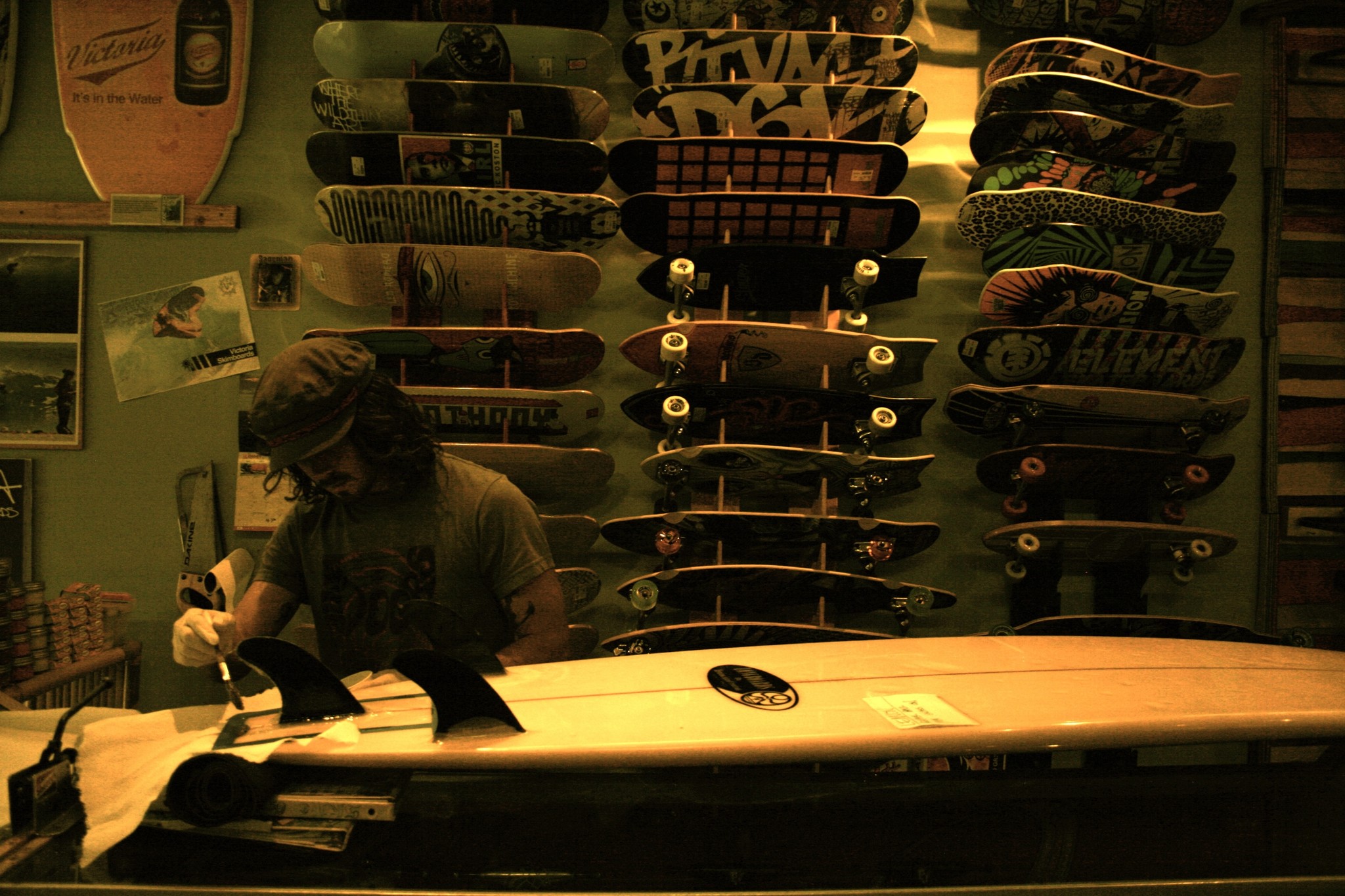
[247,333,375,474]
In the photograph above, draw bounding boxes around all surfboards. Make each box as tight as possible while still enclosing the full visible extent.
[206,628,1345,782]
[47,0,256,211]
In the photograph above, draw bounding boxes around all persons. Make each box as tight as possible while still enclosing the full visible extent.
[170,334,573,670]
[54,370,77,435]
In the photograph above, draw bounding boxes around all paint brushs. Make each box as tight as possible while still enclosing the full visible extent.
[204,610,244,710]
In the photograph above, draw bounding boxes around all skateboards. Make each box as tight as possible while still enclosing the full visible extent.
[293,0,1273,661]
